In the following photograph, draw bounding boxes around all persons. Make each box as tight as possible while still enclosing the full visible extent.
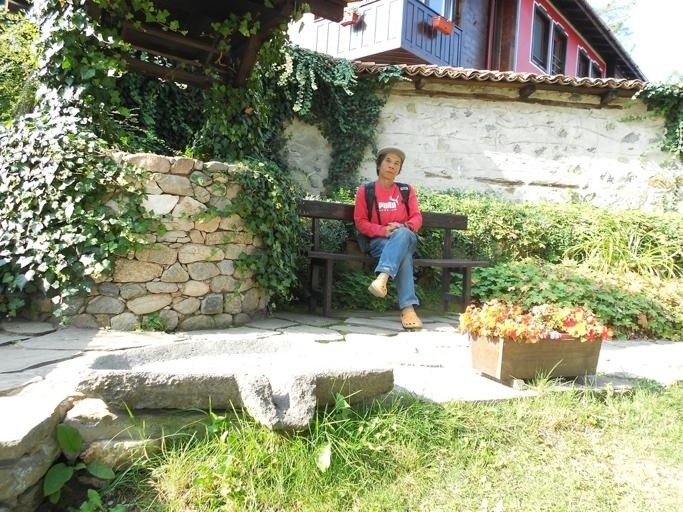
[354,147,424,329]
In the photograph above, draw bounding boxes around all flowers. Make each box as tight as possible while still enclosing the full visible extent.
[457,299,613,345]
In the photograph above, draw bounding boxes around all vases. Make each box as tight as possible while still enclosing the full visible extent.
[470,331,605,380]
[432,16,453,35]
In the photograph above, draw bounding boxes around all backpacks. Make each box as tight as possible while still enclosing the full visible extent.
[358,181,409,253]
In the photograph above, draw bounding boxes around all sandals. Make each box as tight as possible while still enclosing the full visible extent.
[400,310,423,328]
[368,275,388,298]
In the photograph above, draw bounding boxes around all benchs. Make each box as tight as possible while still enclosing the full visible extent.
[296,199,489,316]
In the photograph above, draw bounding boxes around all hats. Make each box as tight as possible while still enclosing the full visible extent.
[377,147,405,163]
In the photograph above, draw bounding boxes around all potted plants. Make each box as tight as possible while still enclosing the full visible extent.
[339,7,359,27]
